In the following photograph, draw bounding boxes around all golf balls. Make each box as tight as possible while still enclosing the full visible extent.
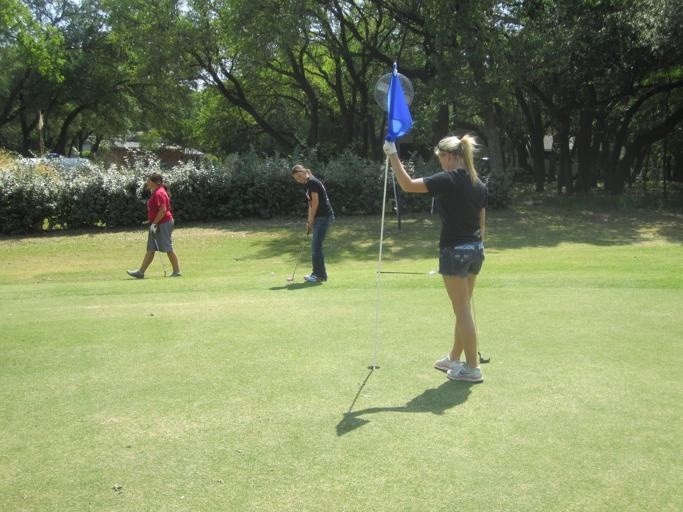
[271,272,273,275]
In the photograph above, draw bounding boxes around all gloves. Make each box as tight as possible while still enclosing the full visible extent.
[382,139,397,154]
[149,224,158,234]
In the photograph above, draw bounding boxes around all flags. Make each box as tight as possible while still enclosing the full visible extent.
[387,68,413,142]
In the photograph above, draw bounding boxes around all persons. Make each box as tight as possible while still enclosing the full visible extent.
[383,135,489,383]
[123,173,182,279]
[291,164,334,282]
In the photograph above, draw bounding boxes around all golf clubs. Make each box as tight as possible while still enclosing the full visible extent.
[286,233,306,281]
[470,292,489,364]
[153,233,166,278]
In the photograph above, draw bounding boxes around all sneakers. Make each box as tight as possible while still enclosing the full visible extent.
[169,272,182,278]
[303,273,325,282]
[448,367,483,383]
[126,270,143,278]
[434,357,462,372]
